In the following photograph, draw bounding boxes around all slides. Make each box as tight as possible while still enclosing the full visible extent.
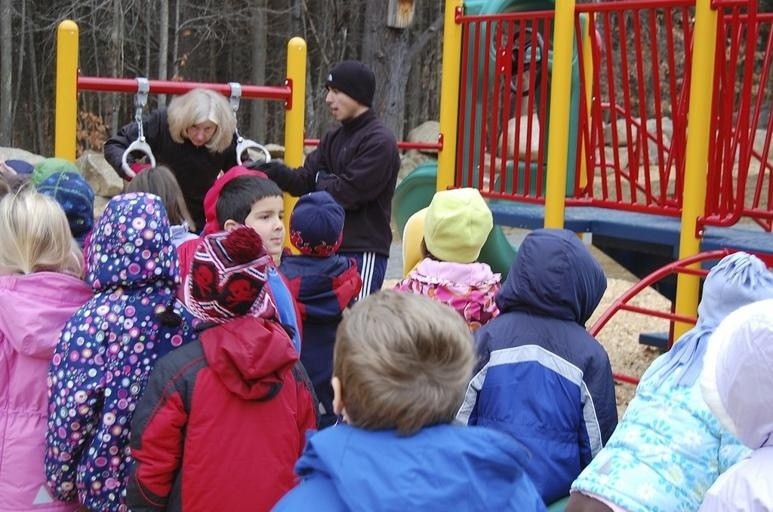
[391,157,544,286]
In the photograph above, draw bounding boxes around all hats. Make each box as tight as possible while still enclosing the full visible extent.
[694,248,772,338]
[182,225,280,325]
[0,155,93,236]
[423,187,495,264]
[324,59,375,108]
[289,191,346,257]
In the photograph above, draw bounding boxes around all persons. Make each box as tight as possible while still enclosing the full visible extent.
[101,87,253,234]
[242,60,400,305]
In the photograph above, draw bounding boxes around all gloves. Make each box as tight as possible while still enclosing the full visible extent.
[123,162,151,182]
